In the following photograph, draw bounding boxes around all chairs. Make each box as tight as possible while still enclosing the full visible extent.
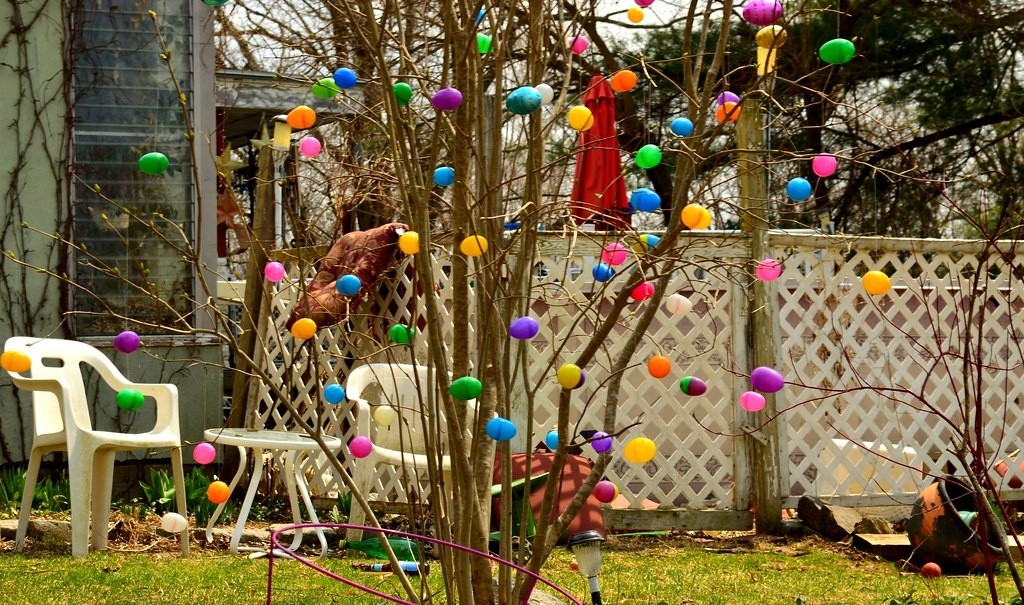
[346,364,499,541]
[3,336,191,558]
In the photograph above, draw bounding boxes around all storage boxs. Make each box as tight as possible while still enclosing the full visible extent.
[817,439,923,494]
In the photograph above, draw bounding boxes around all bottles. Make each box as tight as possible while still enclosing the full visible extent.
[339,537,420,559]
[368,559,430,575]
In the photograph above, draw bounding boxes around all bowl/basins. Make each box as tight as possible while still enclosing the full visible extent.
[907,475,1007,570]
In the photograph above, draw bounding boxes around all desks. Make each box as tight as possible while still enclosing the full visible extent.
[204,428,341,560]
